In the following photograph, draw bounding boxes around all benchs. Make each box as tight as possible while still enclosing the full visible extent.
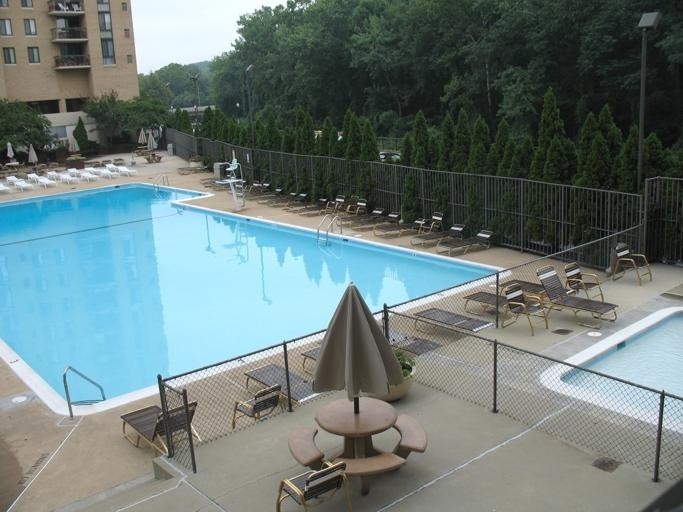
[242,289,509,404]
[0,144,163,195]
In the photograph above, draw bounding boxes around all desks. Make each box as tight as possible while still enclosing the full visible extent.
[314,397,399,458]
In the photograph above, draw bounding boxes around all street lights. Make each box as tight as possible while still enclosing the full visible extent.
[246,63,255,122]
[636,12,663,189]
[192,73,201,113]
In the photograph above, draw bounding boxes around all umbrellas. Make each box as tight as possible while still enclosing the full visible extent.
[310,279,405,414]
[6,142,13,162]
[147,131,157,153]
[138,127,147,144]
[27,144,38,167]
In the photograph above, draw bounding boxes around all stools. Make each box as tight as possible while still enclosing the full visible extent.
[288,414,428,494]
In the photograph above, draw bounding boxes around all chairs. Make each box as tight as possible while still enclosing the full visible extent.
[231,383,285,430]
[501,241,653,335]
[274,459,353,511]
[120,399,203,459]
[200,173,494,257]
